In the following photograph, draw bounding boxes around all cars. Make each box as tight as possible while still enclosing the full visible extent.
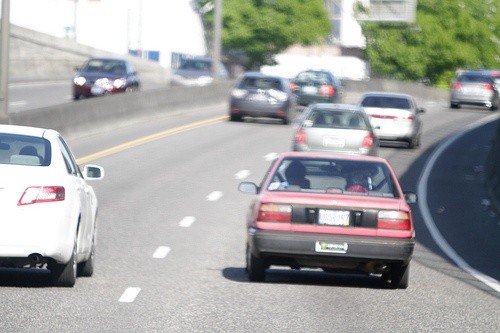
[168,57,229,89]
[0,124,105,288]
[71,57,141,101]
[227,73,298,124]
[450,69,500,111]
[288,70,344,106]
[358,92,426,149]
[237,151,418,290]
[292,101,380,159]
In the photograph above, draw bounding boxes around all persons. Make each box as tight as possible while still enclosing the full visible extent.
[284,160,306,189]
[19,146,43,164]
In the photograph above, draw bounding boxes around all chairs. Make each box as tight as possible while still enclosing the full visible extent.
[285,160,311,189]
[349,116,359,126]
[245,79,254,86]
[315,113,326,124]
[345,172,369,190]
[271,81,281,88]
[20,146,37,155]
